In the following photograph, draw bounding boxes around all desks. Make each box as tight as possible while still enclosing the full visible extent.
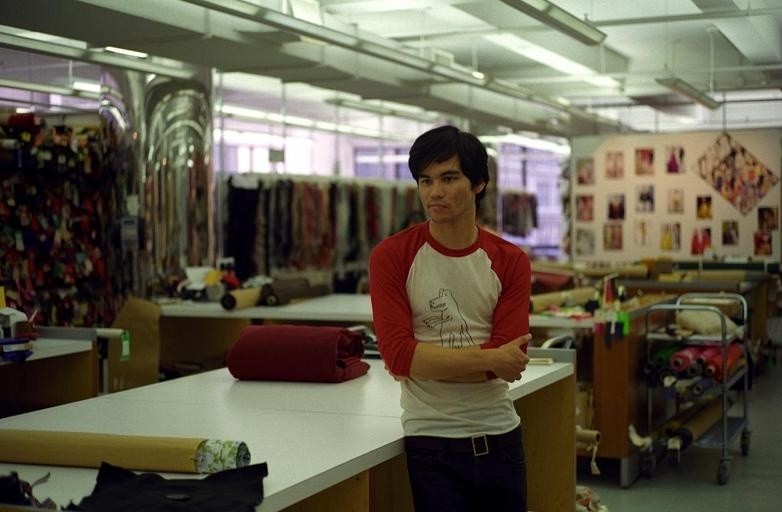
[147,292,595,371]
[533,288,679,489]
[1,325,121,416]
[0,342,578,512]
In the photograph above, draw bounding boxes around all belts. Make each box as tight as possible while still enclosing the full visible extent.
[404,425,521,457]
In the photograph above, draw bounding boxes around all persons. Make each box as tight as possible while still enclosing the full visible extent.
[368,125,533,511]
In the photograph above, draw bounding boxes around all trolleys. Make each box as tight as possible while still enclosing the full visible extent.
[646,288,756,487]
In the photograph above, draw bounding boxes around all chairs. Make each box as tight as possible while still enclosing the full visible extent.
[100,297,160,392]
